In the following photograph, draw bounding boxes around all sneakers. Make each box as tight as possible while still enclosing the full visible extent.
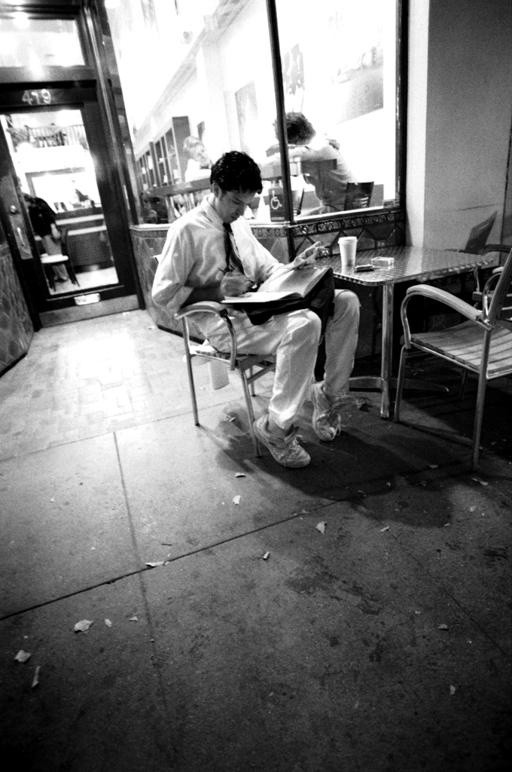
[255,414,311,468]
[310,382,341,441]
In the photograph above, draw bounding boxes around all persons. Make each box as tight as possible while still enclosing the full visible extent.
[150,150,363,470]
[264,110,364,216]
[20,189,80,293]
[178,134,214,202]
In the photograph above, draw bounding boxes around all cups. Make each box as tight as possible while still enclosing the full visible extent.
[337,234,358,266]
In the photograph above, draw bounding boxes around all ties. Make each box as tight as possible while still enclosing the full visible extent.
[221,223,246,279]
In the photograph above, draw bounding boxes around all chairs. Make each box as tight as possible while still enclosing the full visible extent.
[325,180,374,213]
[147,253,340,455]
[292,188,307,218]
[459,209,497,253]
[40,226,84,292]
[391,234,511,471]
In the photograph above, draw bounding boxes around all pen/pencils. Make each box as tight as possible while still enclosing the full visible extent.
[216,266,226,274]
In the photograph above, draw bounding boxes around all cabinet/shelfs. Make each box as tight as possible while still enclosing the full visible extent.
[136,142,165,191]
[152,116,194,186]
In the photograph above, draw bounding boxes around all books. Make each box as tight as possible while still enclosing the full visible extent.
[218,266,331,306]
